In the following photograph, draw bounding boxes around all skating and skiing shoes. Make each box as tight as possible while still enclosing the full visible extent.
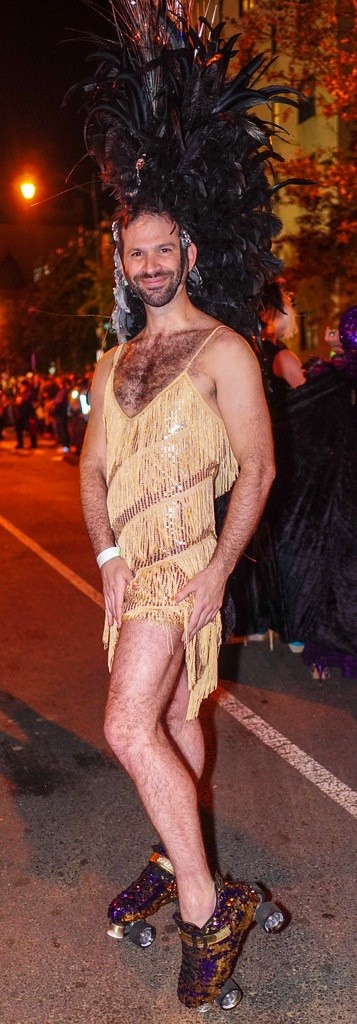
[106,841,180,948]
[173,877,286,1012]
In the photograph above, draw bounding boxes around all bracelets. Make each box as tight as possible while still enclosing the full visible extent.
[96,546,121,569]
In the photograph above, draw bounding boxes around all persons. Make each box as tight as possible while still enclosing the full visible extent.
[0,311,357,679]
[79,191,289,1012]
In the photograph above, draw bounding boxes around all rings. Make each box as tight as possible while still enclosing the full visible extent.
[329,329,335,336]
